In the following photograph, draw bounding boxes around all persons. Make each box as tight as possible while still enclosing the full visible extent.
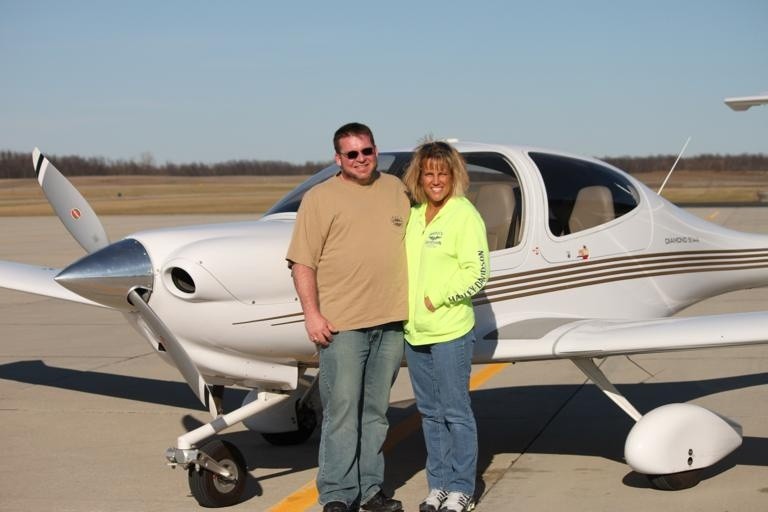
[401,134,491,512]
[284,123,424,512]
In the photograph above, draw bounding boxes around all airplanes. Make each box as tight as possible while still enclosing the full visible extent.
[0,95,768,508]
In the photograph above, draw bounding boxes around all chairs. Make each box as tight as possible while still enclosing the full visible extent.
[475,183,520,251]
[560,185,615,236]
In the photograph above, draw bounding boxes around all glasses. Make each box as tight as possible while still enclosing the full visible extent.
[339,147,372,159]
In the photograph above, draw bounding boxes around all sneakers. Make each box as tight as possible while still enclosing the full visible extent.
[419,489,475,512]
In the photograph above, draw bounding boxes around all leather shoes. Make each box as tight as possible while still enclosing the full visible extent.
[322,489,402,512]
